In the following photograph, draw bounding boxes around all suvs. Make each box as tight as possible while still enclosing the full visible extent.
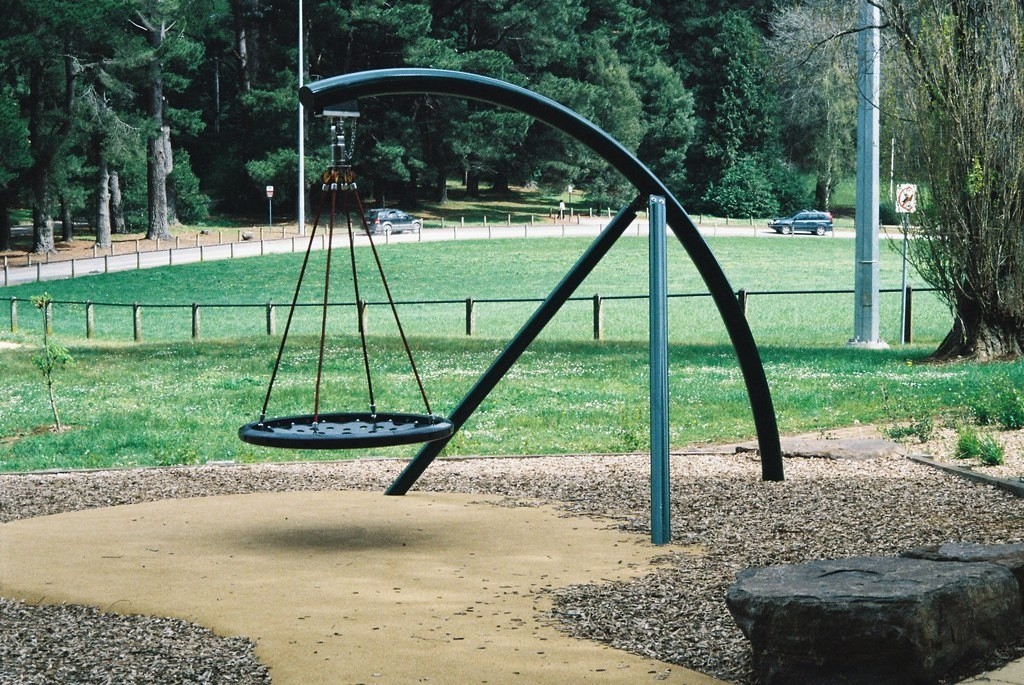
[767,210,834,236]
[360,208,422,236]
